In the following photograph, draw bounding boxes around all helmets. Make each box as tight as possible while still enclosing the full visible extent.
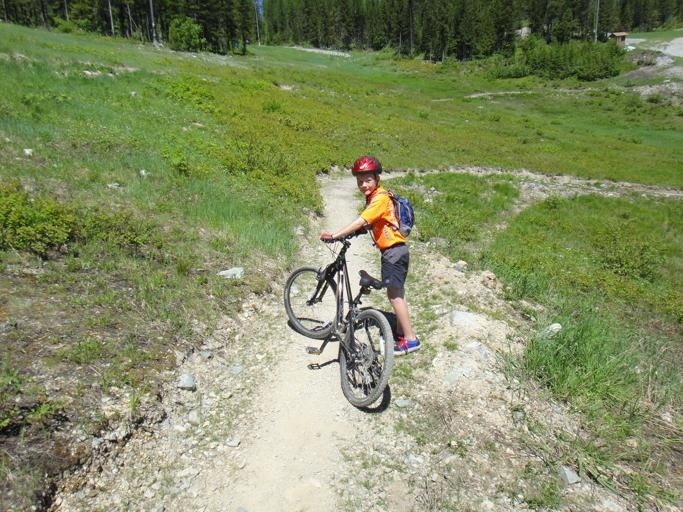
[352,155,382,176]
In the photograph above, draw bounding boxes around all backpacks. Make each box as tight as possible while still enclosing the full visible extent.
[386,190,414,237]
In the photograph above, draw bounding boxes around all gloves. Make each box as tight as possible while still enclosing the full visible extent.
[320,233,334,243]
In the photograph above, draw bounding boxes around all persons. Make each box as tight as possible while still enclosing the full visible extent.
[319,156,421,356]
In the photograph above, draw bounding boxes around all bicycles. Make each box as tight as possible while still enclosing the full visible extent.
[281,227,396,408]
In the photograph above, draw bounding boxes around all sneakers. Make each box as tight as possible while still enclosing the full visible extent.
[381,331,403,342]
[392,336,421,356]
